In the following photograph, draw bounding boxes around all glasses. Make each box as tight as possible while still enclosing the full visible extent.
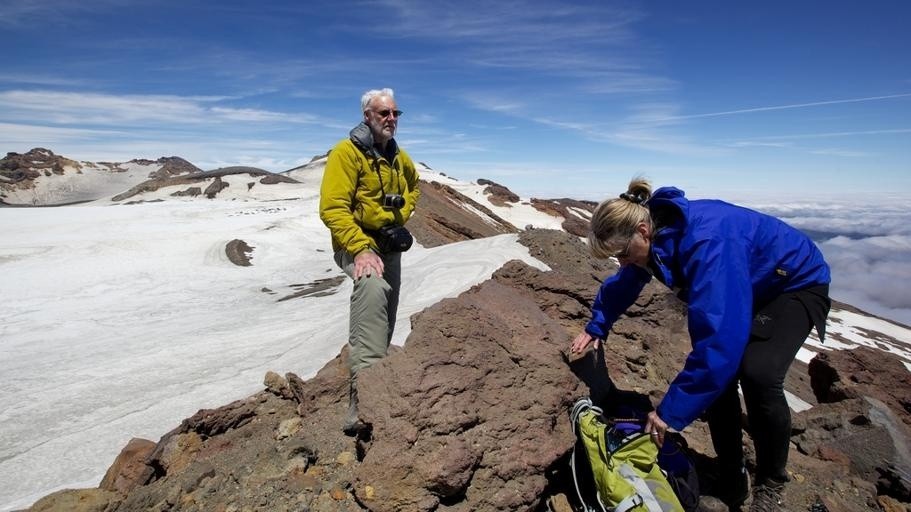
[611,238,632,258]
[378,110,404,116]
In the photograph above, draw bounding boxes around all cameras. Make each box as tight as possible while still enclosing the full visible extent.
[382,194,405,209]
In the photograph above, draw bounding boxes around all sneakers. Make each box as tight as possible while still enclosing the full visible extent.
[748,476,783,511]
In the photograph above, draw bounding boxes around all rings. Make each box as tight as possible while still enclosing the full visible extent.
[651,431,659,437]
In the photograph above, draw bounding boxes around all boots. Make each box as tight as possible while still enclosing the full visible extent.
[343,391,369,431]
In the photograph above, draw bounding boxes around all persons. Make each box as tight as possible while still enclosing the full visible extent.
[319,88,420,435]
[571,169,831,502]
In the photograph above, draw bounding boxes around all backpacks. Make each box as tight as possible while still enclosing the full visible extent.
[563,399,699,512]
